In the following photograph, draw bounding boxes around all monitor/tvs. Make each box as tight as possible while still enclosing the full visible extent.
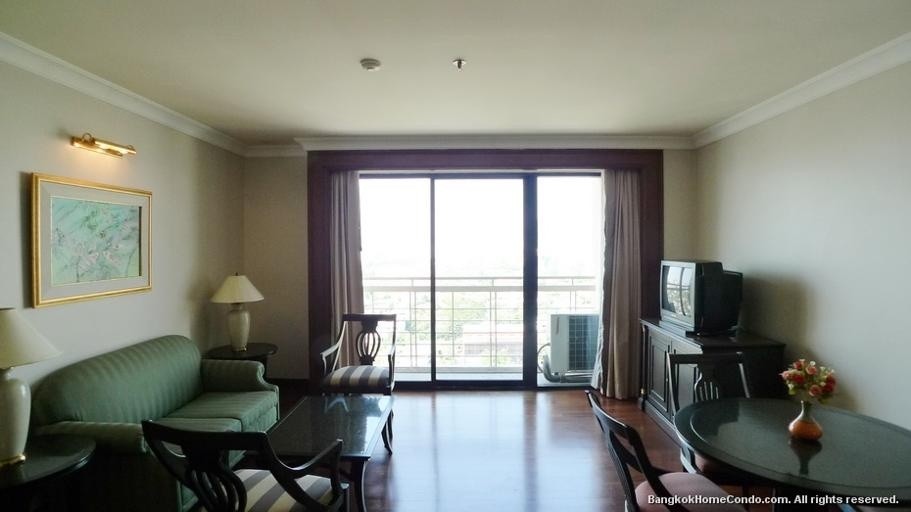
[659,260,743,337]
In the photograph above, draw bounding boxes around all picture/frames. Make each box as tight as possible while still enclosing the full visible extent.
[27,171,156,308]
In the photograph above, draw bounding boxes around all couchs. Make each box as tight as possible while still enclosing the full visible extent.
[26,330,286,511]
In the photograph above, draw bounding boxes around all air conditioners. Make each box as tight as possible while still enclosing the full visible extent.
[547,310,604,374]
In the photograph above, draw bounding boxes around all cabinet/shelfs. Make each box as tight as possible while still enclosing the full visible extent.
[635,317,789,454]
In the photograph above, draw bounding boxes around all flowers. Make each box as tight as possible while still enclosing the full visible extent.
[780,354,841,408]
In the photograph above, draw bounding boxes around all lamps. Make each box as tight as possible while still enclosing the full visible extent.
[0,305,68,470]
[71,131,138,161]
[208,270,266,355]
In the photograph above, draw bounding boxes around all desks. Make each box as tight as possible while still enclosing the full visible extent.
[0,430,102,512]
[204,342,280,382]
[672,394,911,512]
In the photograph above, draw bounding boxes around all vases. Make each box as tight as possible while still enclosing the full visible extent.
[786,399,825,441]
[788,436,825,476]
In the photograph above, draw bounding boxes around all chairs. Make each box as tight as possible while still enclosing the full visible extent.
[582,388,748,512]
[137,414,349,512]
[319,312,397,443]
[665,349,780,512]
